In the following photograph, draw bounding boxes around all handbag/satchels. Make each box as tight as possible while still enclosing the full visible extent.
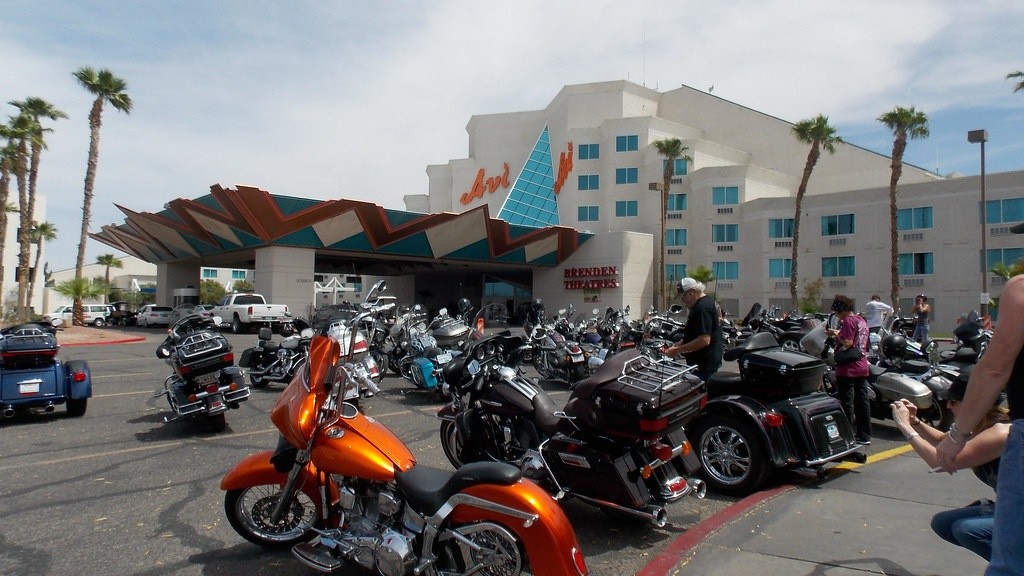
[834,346,863,365]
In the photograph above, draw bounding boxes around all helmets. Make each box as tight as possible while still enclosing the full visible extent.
[883,336,907,358]
[458,299,472,312]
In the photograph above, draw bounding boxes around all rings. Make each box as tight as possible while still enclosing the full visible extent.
[939,459,944,462]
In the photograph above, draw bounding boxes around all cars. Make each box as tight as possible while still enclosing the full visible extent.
[187,304,214,320]
[135,303,173,327]
[169,304,194,327]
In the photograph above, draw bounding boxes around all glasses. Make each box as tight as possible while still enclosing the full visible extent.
[680,292,687,300]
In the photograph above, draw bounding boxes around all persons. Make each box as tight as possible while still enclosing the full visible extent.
[865,294,892,334]
[890,221,1024,576]
[559,320,573,336]
[104,307,107,311]
[117,306,120,311]
[665,278,723,382]
[824,294,870,445]
[66,308,71,311]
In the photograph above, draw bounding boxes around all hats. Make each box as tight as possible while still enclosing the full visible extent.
[672,278,697,300]
[831,294,855,311]
[947,374,969,401]
[872,294,880,301]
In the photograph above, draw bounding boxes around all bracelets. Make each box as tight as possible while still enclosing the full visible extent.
[948,431,966,446]
[949,423,972,441]
[911,420,921,427]
[904,432,919,441]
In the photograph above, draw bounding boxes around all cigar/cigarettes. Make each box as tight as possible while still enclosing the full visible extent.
[928,466,942,473]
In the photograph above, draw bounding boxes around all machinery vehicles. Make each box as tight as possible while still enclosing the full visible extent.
[110,302,138,326]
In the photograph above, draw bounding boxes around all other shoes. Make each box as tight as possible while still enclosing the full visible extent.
[853,434,871,445]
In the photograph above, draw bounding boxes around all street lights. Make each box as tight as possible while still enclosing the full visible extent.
[967,128,989,316]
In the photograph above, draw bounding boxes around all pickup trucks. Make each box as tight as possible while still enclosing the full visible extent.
[207,293,294,334]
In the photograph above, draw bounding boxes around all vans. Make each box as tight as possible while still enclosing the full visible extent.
[49,304,116,327]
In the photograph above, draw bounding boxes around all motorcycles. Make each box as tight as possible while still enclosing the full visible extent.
[152,303,250,433]
[0,317,93,419]
[520,297,845,388]
[800,312,950,434]
[872,314,976,433]
[608,304,868,498]
[866,325,925,362]
[239,279,520,414]
[938,309,995,374]
[220,303,588,576]
[437,329,707,534]
